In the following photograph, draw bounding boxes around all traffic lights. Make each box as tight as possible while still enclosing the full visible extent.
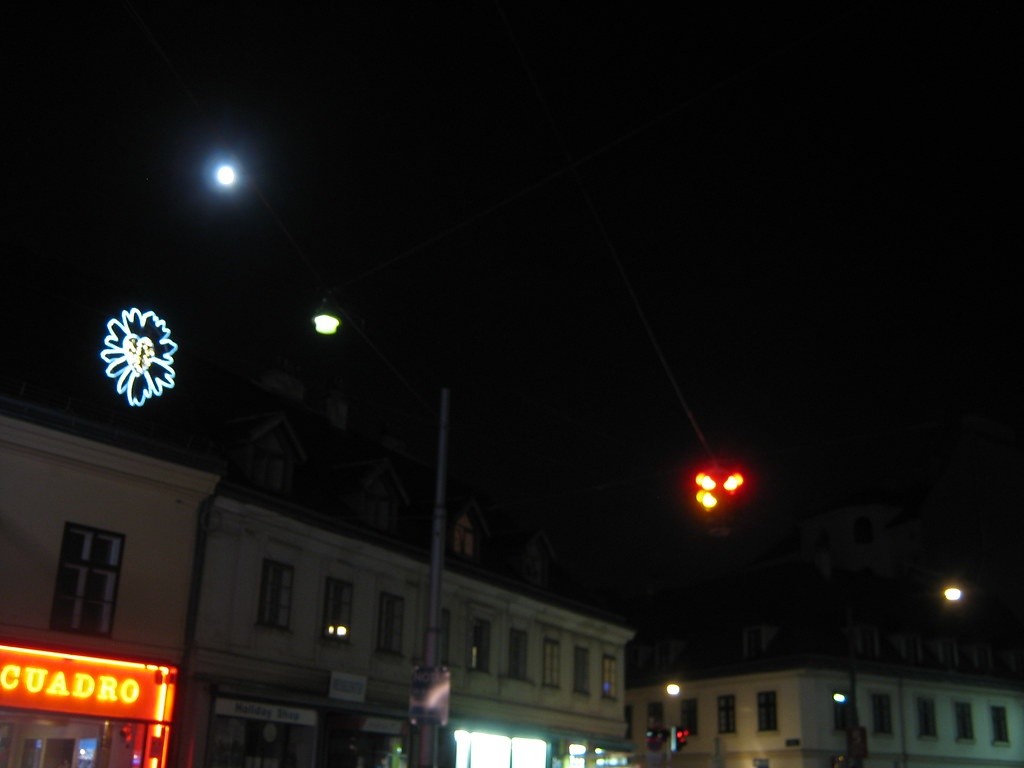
[670,725,690,754]
